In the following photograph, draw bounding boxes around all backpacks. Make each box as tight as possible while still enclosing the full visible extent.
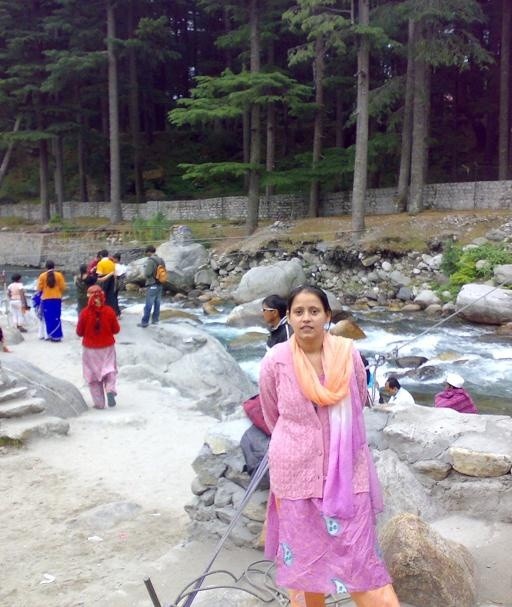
[151,257,167,283]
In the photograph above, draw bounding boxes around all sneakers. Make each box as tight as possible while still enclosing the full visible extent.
[107,392,115,406]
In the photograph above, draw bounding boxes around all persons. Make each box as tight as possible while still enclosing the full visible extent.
[261,293,477,414]
[0,244,166,410]
[258,284,400,607]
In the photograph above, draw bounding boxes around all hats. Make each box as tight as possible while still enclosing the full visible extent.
[446,375,465,388]
